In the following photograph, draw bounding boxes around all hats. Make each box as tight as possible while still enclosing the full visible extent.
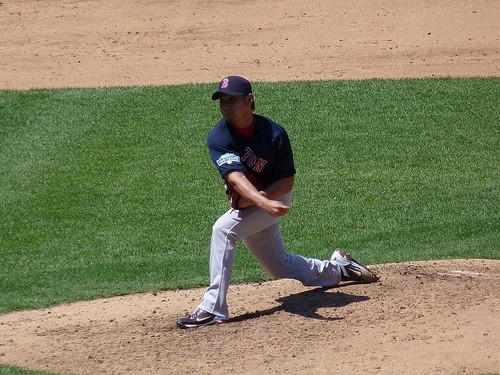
[211,76,252,100]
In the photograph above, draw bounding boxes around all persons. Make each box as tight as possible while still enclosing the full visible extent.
[176,77,378,329]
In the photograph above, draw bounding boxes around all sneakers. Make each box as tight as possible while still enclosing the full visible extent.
[330,249,379,282]
[177,308,221,329]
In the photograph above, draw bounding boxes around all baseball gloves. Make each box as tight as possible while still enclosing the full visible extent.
[223,171,262,210]
